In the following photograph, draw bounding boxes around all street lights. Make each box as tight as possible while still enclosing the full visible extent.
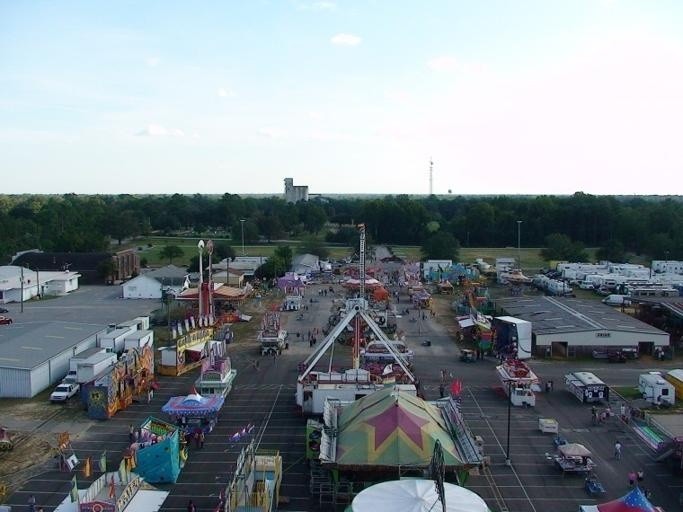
[502,379,518,470]
[18,260,24,314]
[239,219,247,256]
[515,219,522,266]
[416,301,427,337]
[428,159,434,193]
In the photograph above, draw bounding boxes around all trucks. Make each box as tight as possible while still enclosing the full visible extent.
[532,256,683,306]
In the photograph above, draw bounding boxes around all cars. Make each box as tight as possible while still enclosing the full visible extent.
[62,368,79,383]
[0,315,12,326]
[0,307,9,314]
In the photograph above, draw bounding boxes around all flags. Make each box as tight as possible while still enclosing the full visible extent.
[229,431,240,443]
[68,452,134,504]
[238,428,246,437]
[244,423,253,434]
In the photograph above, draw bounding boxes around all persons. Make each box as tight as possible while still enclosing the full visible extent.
[27,493,36,509]
[591,404,611,423]
[397,293,400,303]
[130,429,137,442]
[439,368,453,384]
[619,446,625,464]
[297,326,321,347]
[127,424,133,440]
[630,408,635,420]
[186,499,196,511]
[284,337,288,350]
[439,384,444,398]
[614,441,621,456]
[392,293,396,304]
[36,506,42,512]
[551,379,553,389]
[199,432,204,447]
[637,469,644,483]
[316,287,327,296]
[629,471,636,488]
[656,394,662,410]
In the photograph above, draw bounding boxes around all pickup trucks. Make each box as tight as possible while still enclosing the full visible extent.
[48,382,81,403]
